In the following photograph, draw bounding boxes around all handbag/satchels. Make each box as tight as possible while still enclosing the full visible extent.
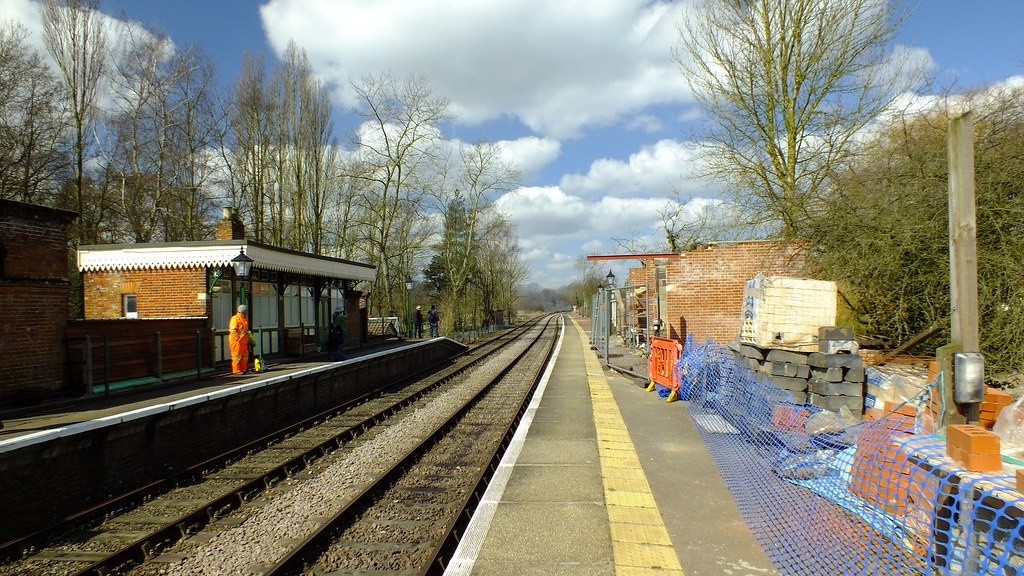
[329,324,334,330]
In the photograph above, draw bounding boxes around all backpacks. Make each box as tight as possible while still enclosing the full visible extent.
[428,310,434,321]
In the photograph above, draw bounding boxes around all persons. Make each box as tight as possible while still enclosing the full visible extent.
[413,306,423,339]
[333,306,347,352]
[229,305,251,375]
[428,305,441,338]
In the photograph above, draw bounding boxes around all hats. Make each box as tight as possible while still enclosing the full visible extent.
[335,305,344,312]
[238,305,247,313]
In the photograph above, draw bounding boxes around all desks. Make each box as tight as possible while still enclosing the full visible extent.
[892,431,1024,576]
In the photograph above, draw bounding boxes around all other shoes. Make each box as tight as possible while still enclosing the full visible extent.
[437,336,440,337]
[237,371,244,375]
[419,337,423,339]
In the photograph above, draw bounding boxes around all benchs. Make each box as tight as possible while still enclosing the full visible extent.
[285,325,318,360]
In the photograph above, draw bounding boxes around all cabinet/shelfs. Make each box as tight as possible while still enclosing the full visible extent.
[632,282,649,353]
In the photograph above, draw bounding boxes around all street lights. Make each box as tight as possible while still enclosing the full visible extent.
[606,269,615,336]
[405,276,414,338]
